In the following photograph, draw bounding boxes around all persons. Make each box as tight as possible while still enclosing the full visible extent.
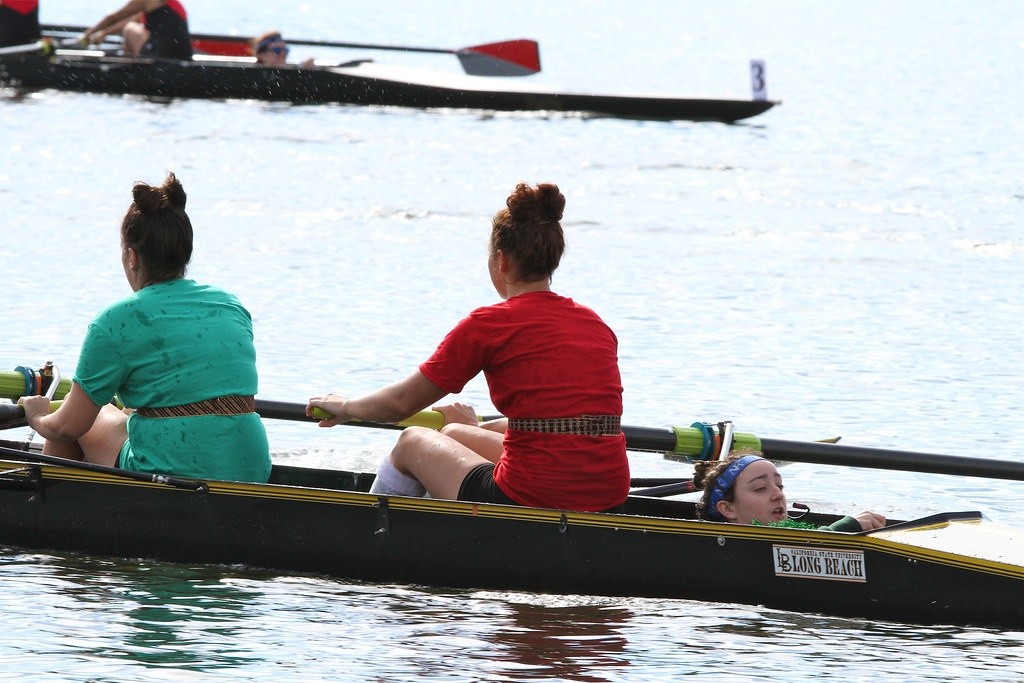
[1,0,315,65]
[304,182,630,512]
[17,171,272,484]
[694,450,886,533]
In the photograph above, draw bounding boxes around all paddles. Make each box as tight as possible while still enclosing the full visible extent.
[25,21,542,81]
[0,367,1023,499]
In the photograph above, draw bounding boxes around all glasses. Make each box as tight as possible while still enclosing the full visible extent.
[266,45,291,54]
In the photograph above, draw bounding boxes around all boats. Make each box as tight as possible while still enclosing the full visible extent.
[2,435,1023,631]
[1,28,783,127]
